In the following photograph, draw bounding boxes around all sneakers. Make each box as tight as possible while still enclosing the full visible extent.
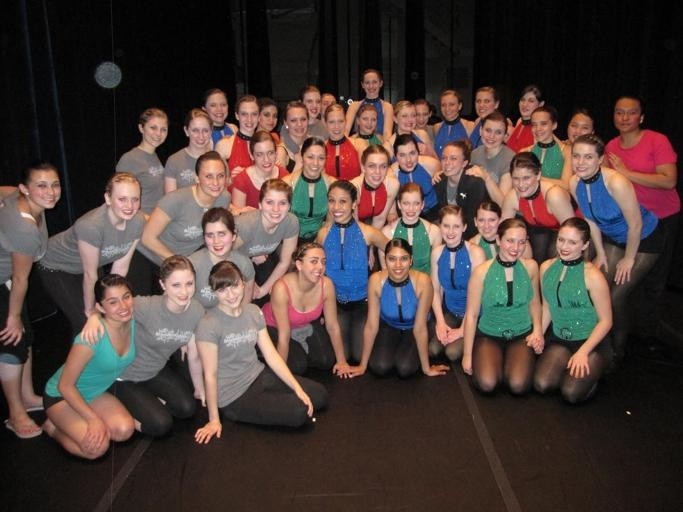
[25,410,48,427]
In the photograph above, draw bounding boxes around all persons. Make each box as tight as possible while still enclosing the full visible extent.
[36,171,144,348]
[343,68,392,141]
[518,104,570,185]
[526,217,615,407]
[335,238,451,384]
[0,157,63,441]
[202,86,238,151]
[193,260,328,445]
[126,151,232,297]
[282,136,340,242]
[499,151,579,302]
[115,107,171,297]
[465,199,499,259]
[248,96,290,168]
[412,100,433,144]
[310,92,336,142]
[376,183,443,275]
[215,94,261,192]
[347,105,392,174]
[604,95,682,367]
[321,104,361,181]
[280,85,328,154]
[459,216,545,398]
[40,273,136,461]
[566,133,667,377]
[230,129,292,211]
[179,205,254,365]
[504,83,546,154]
[349,145,401,271]
[560,99,593,149]
[78,254,208,441]
[388,134,440,227]
[163,107,228,194]
[386,100,440,165]
[424,140,494,241]
[428,205,487,363]
[313,180,389,362]
[466,85,514,148]
[431,89,476,162]
[255,242,352,380]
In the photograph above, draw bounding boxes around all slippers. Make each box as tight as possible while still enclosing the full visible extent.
[26,396,44,412]
[4,418,43,439]
